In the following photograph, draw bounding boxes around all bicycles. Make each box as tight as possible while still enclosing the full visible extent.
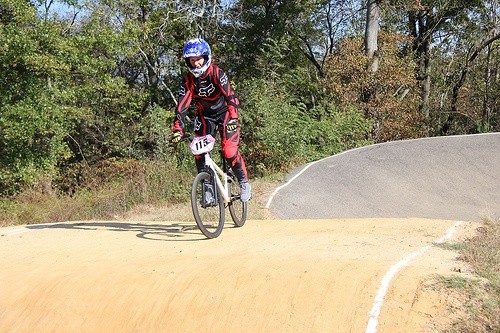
[168,120,252,239]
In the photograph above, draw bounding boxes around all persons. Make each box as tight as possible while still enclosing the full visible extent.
[171,38,251,205]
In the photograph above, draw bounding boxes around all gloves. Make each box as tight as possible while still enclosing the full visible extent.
[226,119,236,131]
[171,131,181,142]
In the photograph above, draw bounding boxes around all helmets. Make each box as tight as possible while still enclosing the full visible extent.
[182,38,212,78]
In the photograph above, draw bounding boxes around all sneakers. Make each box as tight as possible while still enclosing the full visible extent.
[239,183,251,202]
[205,184,214,203]
